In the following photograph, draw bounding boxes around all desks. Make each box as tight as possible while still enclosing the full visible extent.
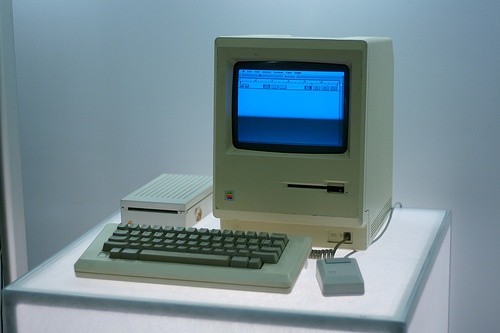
[2,206,454,333]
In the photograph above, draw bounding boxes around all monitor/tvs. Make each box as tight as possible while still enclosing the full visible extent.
[213,35,393,250]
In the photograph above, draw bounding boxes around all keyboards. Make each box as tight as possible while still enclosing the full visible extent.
[73,222,312,293]
